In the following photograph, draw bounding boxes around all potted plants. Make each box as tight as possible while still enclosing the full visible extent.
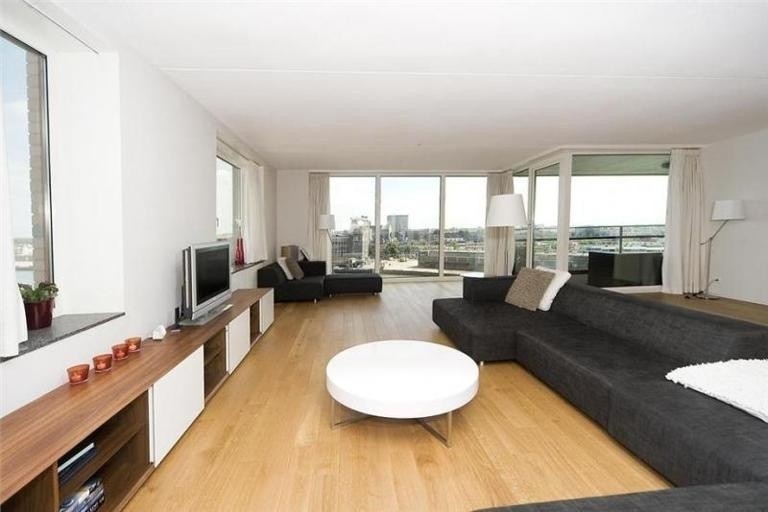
[17,278,59,328]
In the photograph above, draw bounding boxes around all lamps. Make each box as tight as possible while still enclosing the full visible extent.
[481,191,527,274]
[697,198,744,299]
[316,214,336,273]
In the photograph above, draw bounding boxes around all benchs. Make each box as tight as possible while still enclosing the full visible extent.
[325,274,382,299]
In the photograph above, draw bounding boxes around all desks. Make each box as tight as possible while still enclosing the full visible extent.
[324,341,478,449]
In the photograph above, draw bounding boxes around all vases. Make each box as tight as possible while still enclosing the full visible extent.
[234,237,244,265]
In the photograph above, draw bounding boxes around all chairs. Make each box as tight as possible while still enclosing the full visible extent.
[258,258,327,303]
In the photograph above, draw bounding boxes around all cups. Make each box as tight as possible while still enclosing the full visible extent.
[112,336,142,361]
[65,363,90,385]
[92,354,113,373]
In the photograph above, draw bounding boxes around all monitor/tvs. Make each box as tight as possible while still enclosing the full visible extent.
[179,242,233,326]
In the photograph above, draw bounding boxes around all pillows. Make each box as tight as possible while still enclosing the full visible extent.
[505,266,554,312]
[286,255,303,280]
[665,356,768,423]
[534,265,570,312]
[278,257,294,280]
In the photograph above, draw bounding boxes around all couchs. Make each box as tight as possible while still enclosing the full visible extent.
[424,271,768,512]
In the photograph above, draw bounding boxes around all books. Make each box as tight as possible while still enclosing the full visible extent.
[59,442,106,512]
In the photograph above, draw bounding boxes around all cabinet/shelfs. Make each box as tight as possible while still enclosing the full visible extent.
[1,288,275,512]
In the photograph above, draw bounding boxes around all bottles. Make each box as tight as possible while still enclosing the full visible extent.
[236,238,244,264]
